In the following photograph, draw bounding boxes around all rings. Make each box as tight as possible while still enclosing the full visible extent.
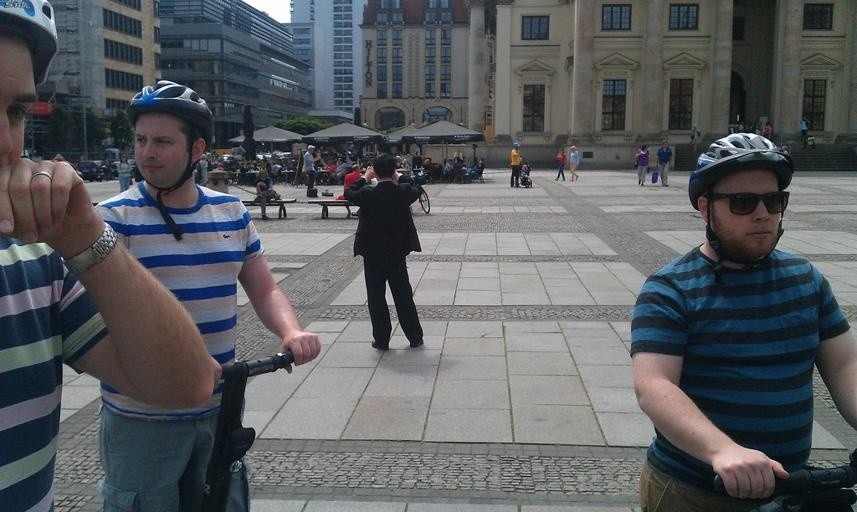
[30,171,53,181]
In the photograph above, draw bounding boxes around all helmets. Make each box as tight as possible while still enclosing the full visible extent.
[688,133,793,211]
[0,0,59,87]
[127,79,214,152]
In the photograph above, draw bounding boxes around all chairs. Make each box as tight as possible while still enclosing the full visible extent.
[239,151,484,185]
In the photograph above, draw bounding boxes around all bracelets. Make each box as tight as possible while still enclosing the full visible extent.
[64,222,118,274]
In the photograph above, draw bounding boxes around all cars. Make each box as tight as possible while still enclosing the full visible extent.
[66,145,141,186]
[212,149,295,169]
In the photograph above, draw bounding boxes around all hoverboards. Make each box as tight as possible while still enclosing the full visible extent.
[199,346,296,512]
[703,445,857,509]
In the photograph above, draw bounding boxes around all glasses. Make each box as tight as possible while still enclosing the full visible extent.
[704,191,790,215]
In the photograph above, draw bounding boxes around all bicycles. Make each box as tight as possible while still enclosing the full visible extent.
[409,180,432,217]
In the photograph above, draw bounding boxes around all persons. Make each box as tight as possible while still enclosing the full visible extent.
[567,144,579,181]
[509,142,523,187]
[73,82,320,512]
[344,153,424,350]
[0,0,224,512]
[116,145,488,219]
[630,131,856,511]
[632,114,817,187]
[554,148,567,181]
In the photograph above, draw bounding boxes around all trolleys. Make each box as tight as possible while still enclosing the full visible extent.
[518,163,533,189]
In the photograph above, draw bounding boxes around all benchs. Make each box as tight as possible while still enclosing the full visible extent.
[239,197,297,221]
[307,198,358,220]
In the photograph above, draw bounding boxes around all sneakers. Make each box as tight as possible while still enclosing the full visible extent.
[410,339,423,347]
[372,339,389,350]
